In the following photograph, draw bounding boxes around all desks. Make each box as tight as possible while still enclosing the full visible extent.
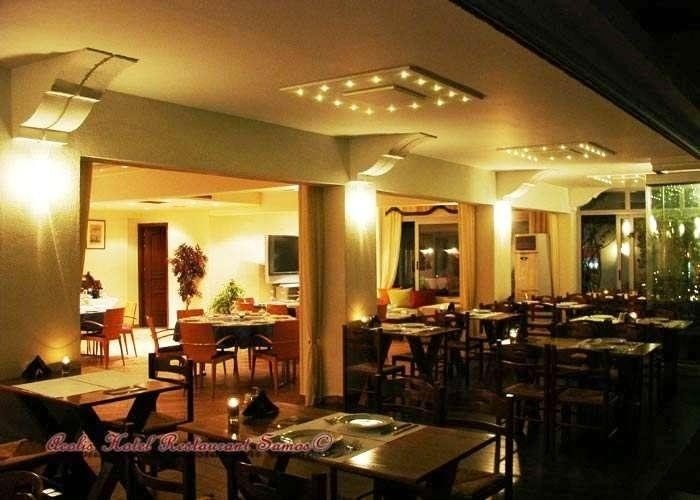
[568,314,694,367]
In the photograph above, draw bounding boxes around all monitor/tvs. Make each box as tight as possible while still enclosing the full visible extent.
[268,235,299,275]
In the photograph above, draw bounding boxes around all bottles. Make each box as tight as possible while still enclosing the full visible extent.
[244,386,259,405]
[369,314,378,328]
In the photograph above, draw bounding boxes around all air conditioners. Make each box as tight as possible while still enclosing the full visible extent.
[512,232,554,303]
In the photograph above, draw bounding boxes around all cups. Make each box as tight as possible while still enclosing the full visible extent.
[225,395,239,424]
[377,304,389,321]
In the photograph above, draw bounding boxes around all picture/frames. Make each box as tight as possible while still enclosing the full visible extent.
[86,219,106,249]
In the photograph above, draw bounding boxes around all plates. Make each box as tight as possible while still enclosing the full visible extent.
[178,314,289,324]
[460,308,490,317]
[279,429,342,447]
[558,302,577,307]
[585,338,625,351]
[384,323,425,333]
[339,414,394,432]
